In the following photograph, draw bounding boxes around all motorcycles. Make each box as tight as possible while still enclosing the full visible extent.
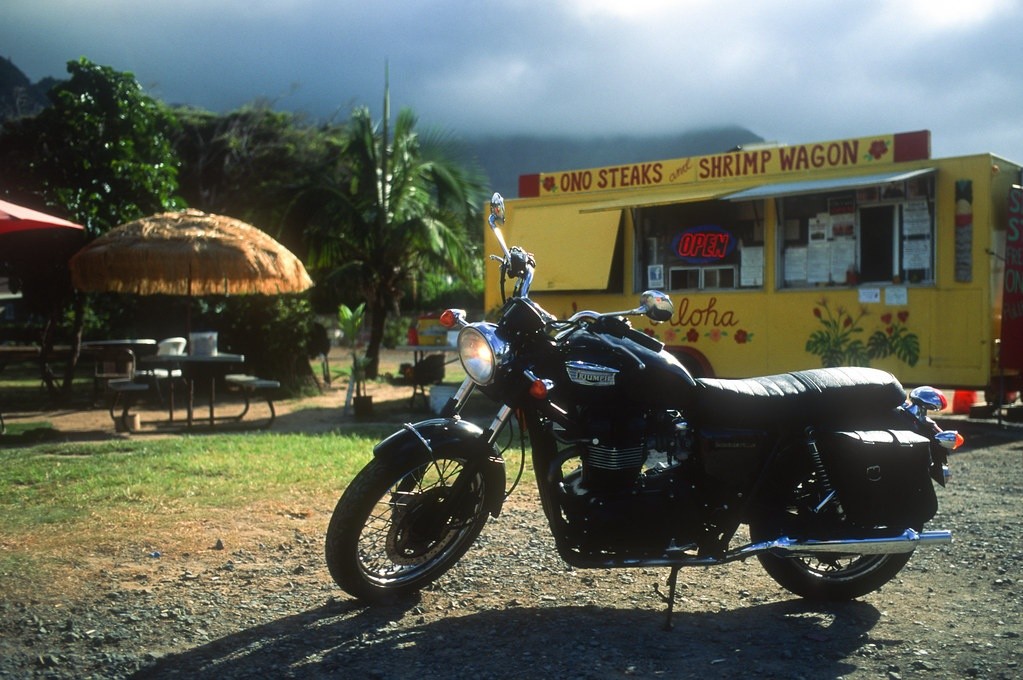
[322,189,968,609]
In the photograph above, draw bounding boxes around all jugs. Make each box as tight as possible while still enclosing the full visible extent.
[94,345,136,384]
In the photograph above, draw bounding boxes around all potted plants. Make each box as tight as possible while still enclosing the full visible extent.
[335,301,373,418]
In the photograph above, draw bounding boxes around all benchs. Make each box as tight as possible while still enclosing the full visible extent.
[108,366,280,425]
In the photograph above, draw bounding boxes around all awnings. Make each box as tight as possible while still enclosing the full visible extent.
[578,166,935,213]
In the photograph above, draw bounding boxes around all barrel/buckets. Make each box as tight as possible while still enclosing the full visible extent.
[415,312,447,346]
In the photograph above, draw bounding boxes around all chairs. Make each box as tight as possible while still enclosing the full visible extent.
[91,349,136,408]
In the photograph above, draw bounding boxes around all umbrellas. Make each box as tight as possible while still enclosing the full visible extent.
[0,200,84,233]
[72,208,314,424]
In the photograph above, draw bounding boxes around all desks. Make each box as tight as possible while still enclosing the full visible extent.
[394,344,458,411]
[144,353,244,429]
